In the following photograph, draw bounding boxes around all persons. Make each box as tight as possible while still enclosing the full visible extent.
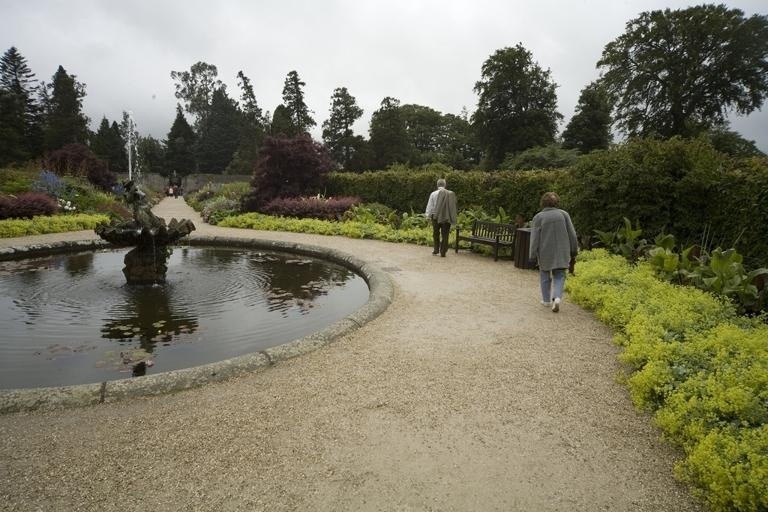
[163,182,184,199]
[529,191,578,313]
[425,178,457,257]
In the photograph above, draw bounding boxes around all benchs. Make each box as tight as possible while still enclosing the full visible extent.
[455,219,517,262]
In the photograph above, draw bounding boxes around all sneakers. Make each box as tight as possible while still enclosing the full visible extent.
[540,300,552,307]
[552,298,561,312]
